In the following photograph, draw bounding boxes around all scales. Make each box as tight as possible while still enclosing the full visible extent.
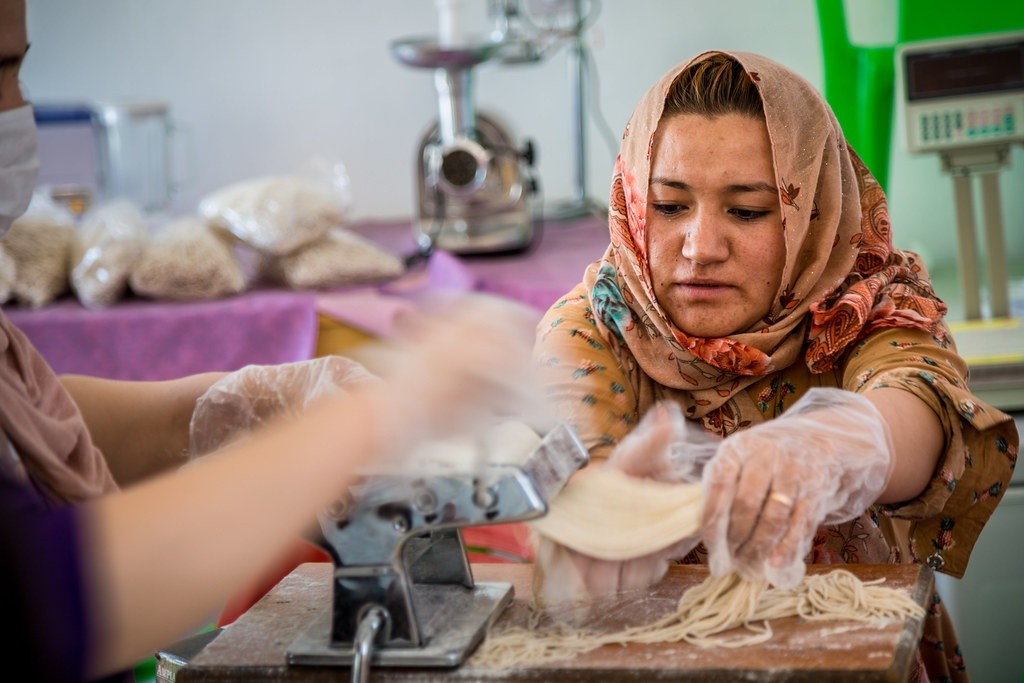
[899,35,1024,410]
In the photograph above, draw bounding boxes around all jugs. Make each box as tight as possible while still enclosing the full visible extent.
[34,105,117,216]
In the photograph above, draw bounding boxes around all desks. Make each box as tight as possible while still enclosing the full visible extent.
[0,209,607,379]
[171,560,935,683]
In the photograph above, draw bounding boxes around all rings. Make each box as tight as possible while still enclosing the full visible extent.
[769,491,794,509]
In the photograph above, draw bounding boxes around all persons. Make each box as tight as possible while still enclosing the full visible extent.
[535,48,968,683]
[0,1,547,683]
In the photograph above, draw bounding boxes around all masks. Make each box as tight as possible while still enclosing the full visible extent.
[0,103,42,239]
